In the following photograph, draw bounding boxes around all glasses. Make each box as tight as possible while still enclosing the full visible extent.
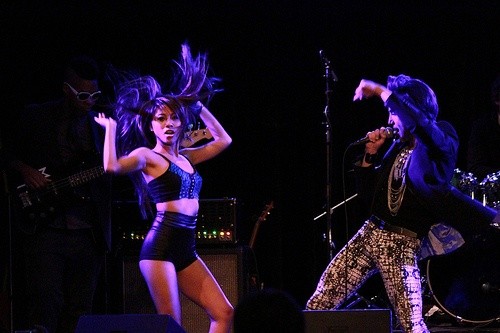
[66,82,101,102]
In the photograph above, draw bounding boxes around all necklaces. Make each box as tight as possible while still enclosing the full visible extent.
[387,143,416,217]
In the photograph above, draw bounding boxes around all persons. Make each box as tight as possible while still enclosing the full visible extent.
[305,79,459,333]
[9,43,307,333]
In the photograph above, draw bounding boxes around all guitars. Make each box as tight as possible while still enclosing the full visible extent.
[248,201,274,248]
[9,122,213,235]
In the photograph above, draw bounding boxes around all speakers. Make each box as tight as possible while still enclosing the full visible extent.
[73,312,190,333]
[122,248,240,332]
[302,308,392,333]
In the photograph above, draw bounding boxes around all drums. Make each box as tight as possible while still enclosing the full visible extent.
[480,170,500,226]
[427,223,500,324]
[448,169,479,200]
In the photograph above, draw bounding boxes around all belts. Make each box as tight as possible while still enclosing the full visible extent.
[370,214,417,237]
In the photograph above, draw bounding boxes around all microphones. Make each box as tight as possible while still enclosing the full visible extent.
[350,127,393,146]
[319,50,340,83]
